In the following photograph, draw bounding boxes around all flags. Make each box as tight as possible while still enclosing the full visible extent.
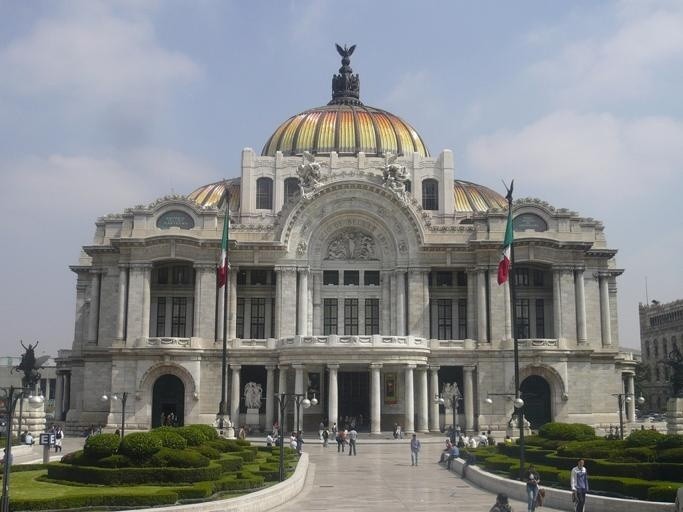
[495,199,514,287]
[214,208,227,290]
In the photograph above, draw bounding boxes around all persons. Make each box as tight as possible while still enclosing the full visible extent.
[570,458,589,512]
[522,463,541,512]
[20,339,39,372]
[244,381,254,409]
[234,414,513,480]
[159,410,179,427]
[608,424,655,441]
[19,421,119,453]
[489,491,513,512]
[442,382,453,408]
[450,381,462,409]
[251,382,263,409]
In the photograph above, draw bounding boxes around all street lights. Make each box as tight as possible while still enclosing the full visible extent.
[483,391,525,480]
[0,384,43,512]
[273,391,318,481]
[434,393,463,445]
[612,393,645,440]
[95,391,132,439]
